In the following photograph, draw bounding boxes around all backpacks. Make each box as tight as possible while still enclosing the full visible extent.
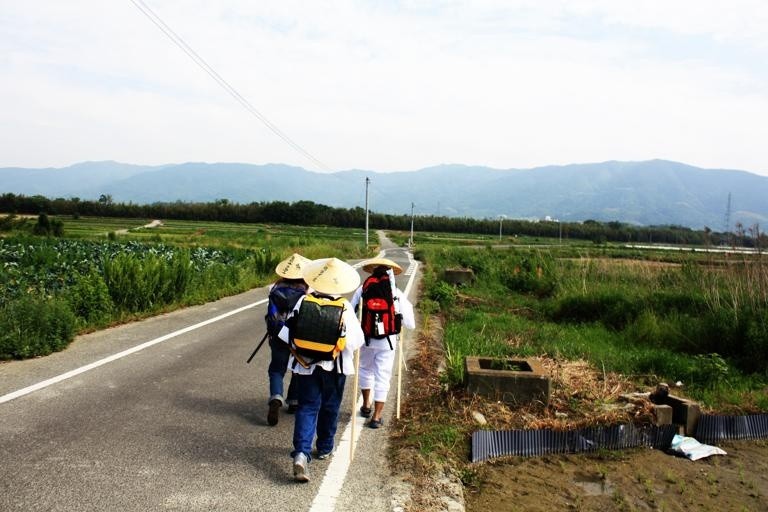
[264,284,305,355]
[362,277,402,340]
[290,294,347,361]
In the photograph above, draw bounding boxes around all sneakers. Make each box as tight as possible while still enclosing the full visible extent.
[292,448,335,481]
[267,399,297,426]
[360,406,384,428]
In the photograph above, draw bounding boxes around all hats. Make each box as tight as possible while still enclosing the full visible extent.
[363,257,401,274]
[274,253,361,295]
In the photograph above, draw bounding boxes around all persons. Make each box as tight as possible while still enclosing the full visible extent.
[265,253,416,481]
